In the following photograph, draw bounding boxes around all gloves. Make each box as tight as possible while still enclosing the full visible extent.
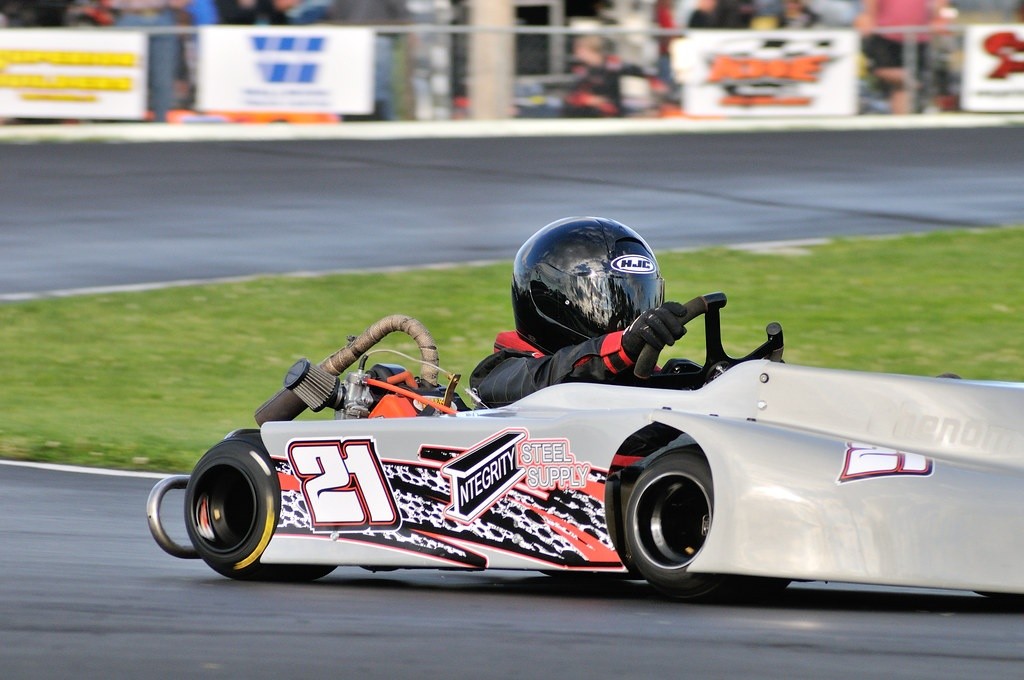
[622,302,688,357]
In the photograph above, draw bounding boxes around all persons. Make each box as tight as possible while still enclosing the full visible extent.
[853,0,955,116]
[651,0,818,55]
[468,215,687,410]
[562,33,671,117]
[0,0,336,27]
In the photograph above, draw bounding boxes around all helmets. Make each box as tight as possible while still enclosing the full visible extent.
[510,214,667,355]
